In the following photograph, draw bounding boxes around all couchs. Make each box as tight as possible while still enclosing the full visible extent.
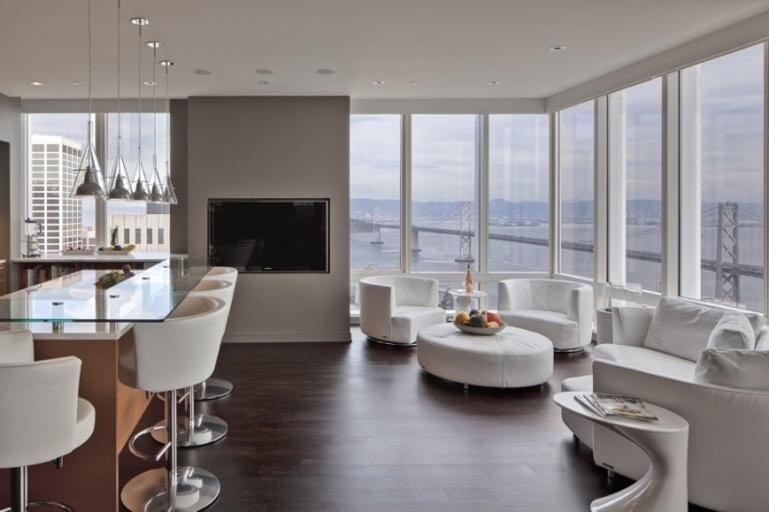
[416,321,554,395]
[496,277,596,356]
[560,301,769,512]
[359,276,441,347]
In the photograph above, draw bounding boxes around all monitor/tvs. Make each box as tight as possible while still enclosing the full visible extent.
[206,197,331,274]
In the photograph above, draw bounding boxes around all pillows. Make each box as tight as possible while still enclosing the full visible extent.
[709,313,756,352]
[691,348,769,392]
[643,297,723,364]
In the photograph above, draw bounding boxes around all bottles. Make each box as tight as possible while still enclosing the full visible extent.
[466,272,472,294]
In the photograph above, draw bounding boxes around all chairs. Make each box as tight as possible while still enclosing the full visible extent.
[120,293,228,512]
[170,266,237,401]
[151,281,234,449]
[1,328,97,512]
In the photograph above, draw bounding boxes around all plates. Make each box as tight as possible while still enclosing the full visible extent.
[98,248,129,255]
[453,321,507,336]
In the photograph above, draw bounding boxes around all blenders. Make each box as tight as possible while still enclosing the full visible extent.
[21,217,42,258]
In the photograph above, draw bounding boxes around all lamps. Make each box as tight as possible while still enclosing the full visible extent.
[130,16,149,202]
[146,40,166,204]
[106,3,135,201]
[68,2,108,199]
[161,59,176,206]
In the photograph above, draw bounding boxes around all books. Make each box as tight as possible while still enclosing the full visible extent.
[574,393,657,421]
